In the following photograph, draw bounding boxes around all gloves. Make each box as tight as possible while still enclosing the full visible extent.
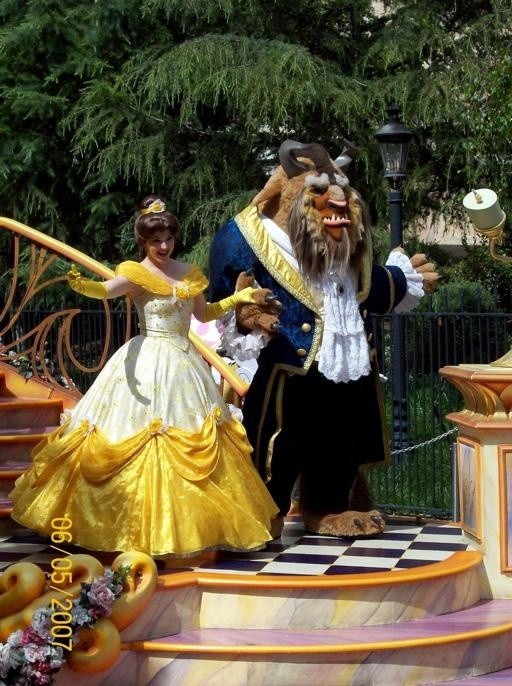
[66,263,108,300]
[204,286,259,322]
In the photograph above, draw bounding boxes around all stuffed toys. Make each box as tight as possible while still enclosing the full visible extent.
[208,141,445,547]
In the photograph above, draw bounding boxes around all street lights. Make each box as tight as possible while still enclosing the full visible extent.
[369,100,418,467]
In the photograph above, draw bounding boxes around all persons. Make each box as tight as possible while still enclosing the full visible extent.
[7,196,285,565]
